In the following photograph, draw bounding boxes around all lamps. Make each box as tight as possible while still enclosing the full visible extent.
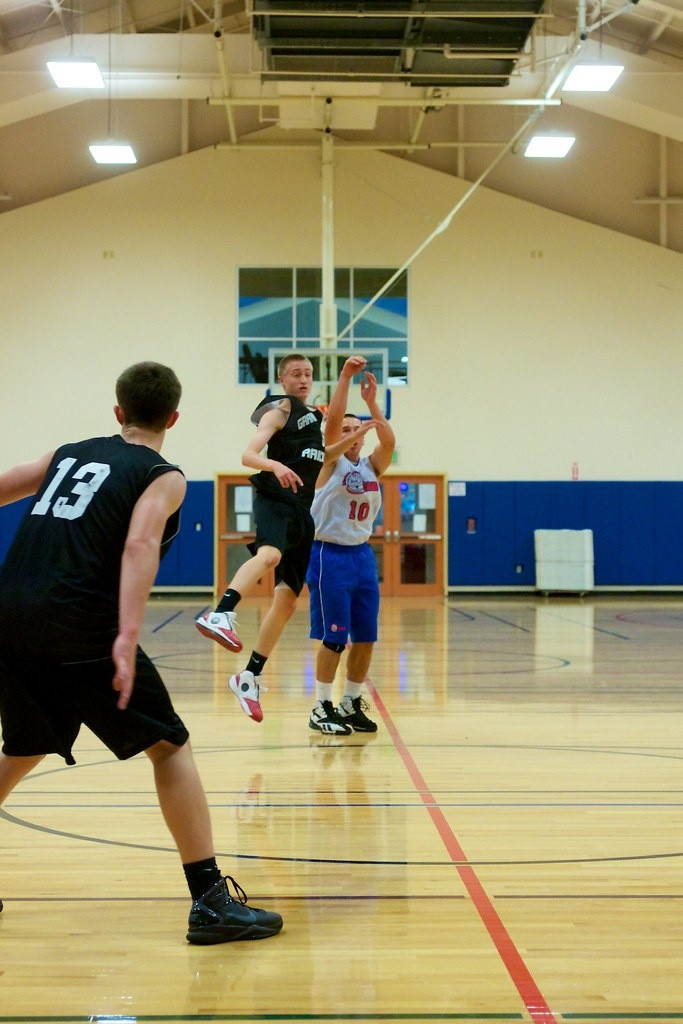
[47,0,104,89]
[88,0,138,165]
[562,0,625,93]
[523,17,577,157]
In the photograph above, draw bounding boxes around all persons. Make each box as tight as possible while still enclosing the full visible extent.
[0,361,283,947]
[306,355,395,736]
[195,353,386,722]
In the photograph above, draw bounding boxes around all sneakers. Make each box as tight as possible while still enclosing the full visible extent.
[229,670,268,723]
[186,875,283,945]
[195,612,243,653]
[309,700,352,735]
[336,695,377,733]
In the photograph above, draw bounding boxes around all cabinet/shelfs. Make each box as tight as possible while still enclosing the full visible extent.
[215,472,448,598]
